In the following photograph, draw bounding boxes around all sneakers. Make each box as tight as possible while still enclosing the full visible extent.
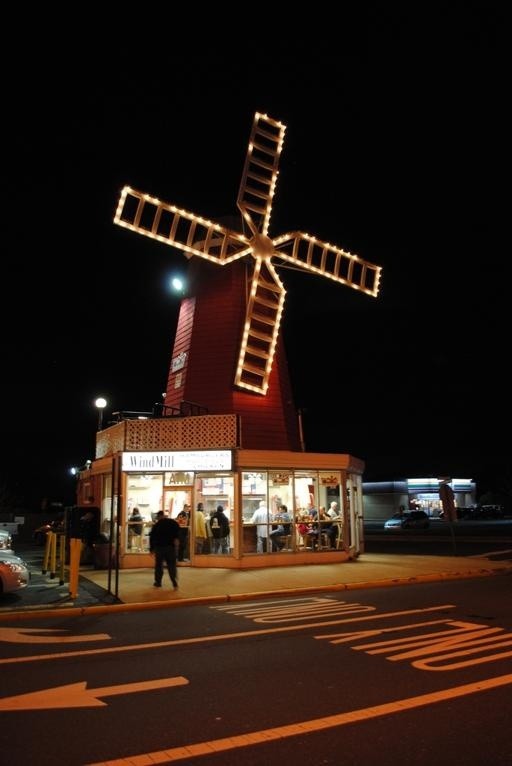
[152,581,179,589]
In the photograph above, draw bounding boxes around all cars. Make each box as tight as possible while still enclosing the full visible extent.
[383,510,429,531]
[0,529,32,593]
[438,504,503,521]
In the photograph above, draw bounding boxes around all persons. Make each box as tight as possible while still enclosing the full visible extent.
[127,501,342,552]
[149,510,180,588]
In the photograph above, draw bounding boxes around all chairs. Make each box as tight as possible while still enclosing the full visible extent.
[272,517,341,552]
[129,524,145,552]
